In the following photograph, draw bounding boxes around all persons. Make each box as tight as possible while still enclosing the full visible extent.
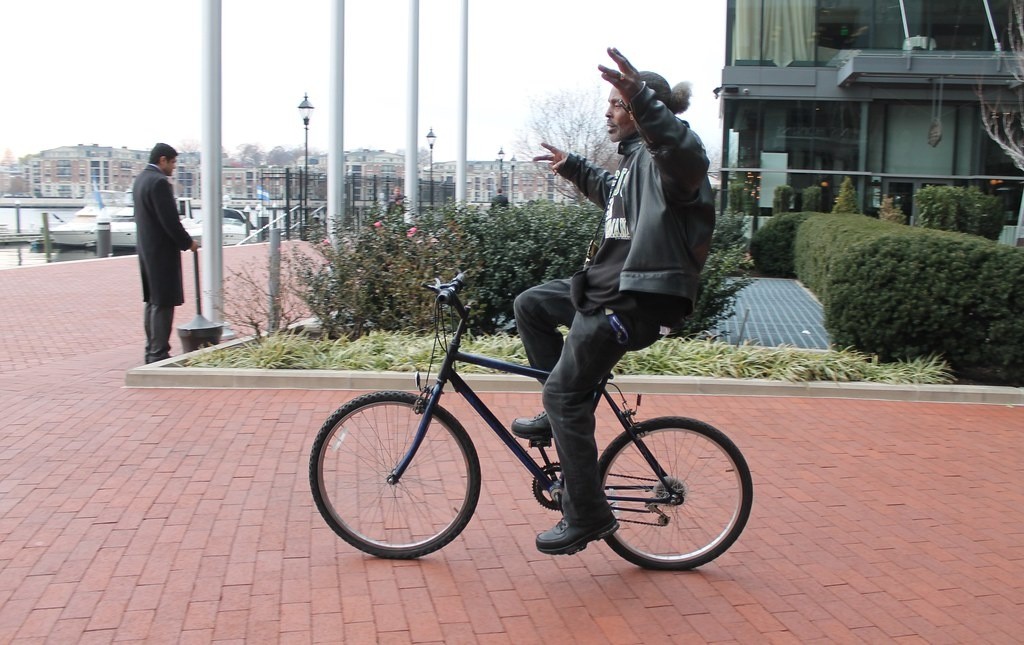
[133,143,198,364]
[510,48,716,554]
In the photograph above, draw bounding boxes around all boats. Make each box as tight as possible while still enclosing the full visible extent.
[46,189,260,250]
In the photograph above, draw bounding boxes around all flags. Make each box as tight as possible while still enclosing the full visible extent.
[257,186,269,201]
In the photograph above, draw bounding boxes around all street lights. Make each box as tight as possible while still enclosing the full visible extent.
[297,93,315,231]
[426,128,437,211]
[497,148,505,189]
[510,156,517,203]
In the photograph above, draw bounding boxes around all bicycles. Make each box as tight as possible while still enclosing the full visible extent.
[308,273,754,572]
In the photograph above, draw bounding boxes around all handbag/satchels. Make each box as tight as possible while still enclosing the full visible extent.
[583,240,599,266]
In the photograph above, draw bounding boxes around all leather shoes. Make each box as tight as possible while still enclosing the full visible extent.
[536,512,621,556]
[511,408,553,440]
